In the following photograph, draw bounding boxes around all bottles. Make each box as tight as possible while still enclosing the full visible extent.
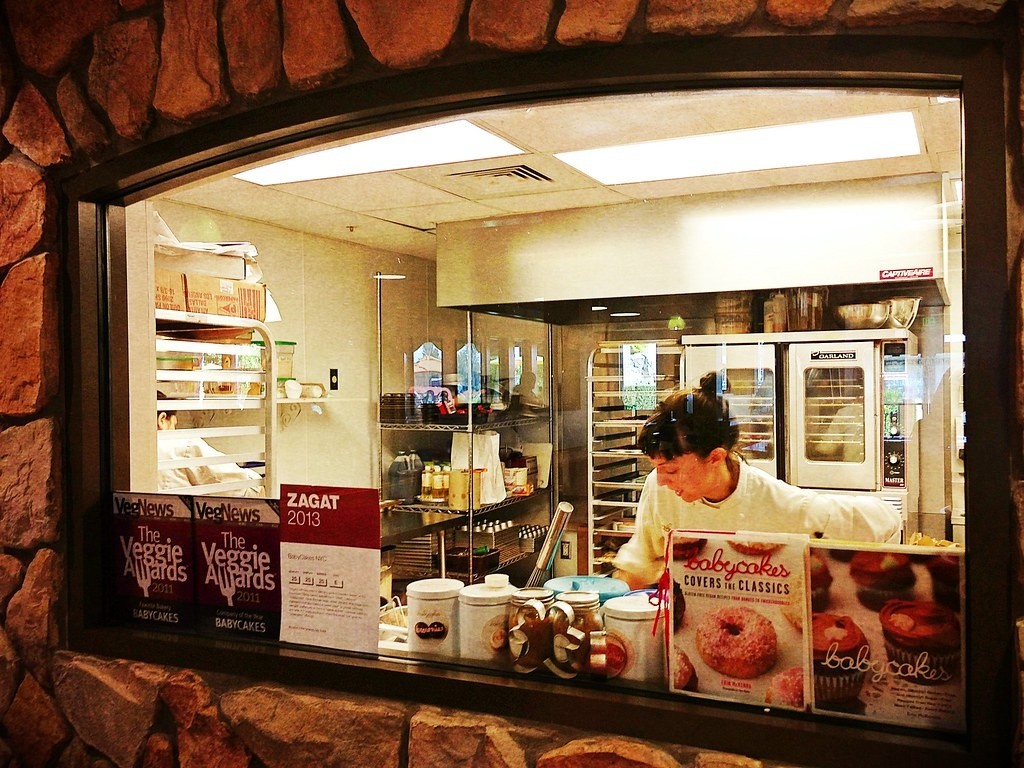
[421,465,450,502]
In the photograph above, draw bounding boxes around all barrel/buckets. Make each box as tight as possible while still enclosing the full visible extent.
[449,470,481,510]
[602,597,669,684]
[406,578,465,657]
[544,575,629,610]
[458,583,518,665]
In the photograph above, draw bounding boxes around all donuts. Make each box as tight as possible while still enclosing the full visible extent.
[673,538,805,708]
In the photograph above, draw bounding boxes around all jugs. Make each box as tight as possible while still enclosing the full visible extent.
[388,450,424,504]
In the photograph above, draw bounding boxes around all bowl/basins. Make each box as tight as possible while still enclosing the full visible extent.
[829,296,924,329]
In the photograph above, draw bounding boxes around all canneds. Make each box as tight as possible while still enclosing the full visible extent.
[509,587,604,675]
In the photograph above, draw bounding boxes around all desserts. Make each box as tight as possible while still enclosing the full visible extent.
[806,549,961,707]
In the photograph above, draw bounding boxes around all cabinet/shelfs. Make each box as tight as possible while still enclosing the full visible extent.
[376,415,548,585]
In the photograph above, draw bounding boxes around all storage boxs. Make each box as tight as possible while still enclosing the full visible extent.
[435,524,547,571]
[155,243,266,323]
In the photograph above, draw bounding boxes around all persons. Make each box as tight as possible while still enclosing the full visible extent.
[597,371,904,627]
[156,388,264,498]
